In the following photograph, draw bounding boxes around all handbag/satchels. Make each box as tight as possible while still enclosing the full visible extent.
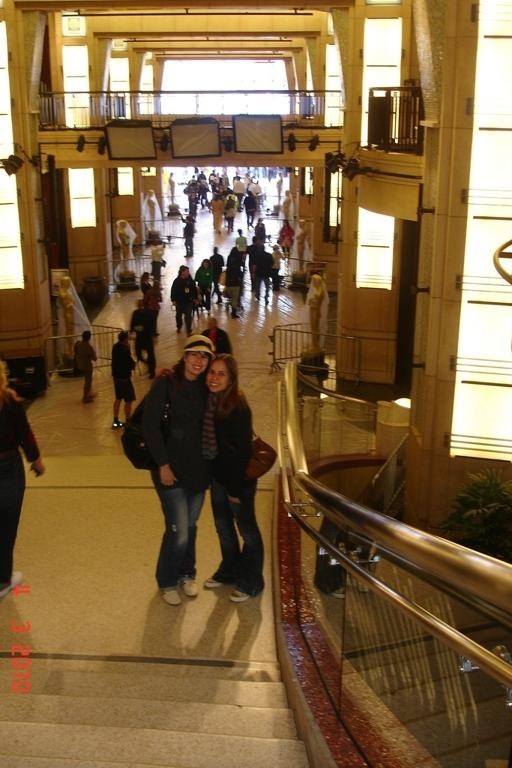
[119,367,179,472]
[244,429,278,484]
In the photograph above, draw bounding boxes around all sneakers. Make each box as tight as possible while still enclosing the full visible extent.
[178,574,201,598]
[202,576,224,590]
[228,588,250,602]
[0,569,26,597]
[161,586,183,607]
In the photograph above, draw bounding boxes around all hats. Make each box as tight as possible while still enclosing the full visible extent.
[183,334,217,360]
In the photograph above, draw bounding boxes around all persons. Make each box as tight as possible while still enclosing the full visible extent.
[308,274,325,347]
[295,218,307,272]
[140,334,217,606]
[201,318,231,355]
[140,167,295,338]
[75,330,98,403]
[58,276,76,353]
[110,331,138,429]
[117,220,131,272]
[0,361,46,599]
[128,299,157,379]
[196,352,265,603]
